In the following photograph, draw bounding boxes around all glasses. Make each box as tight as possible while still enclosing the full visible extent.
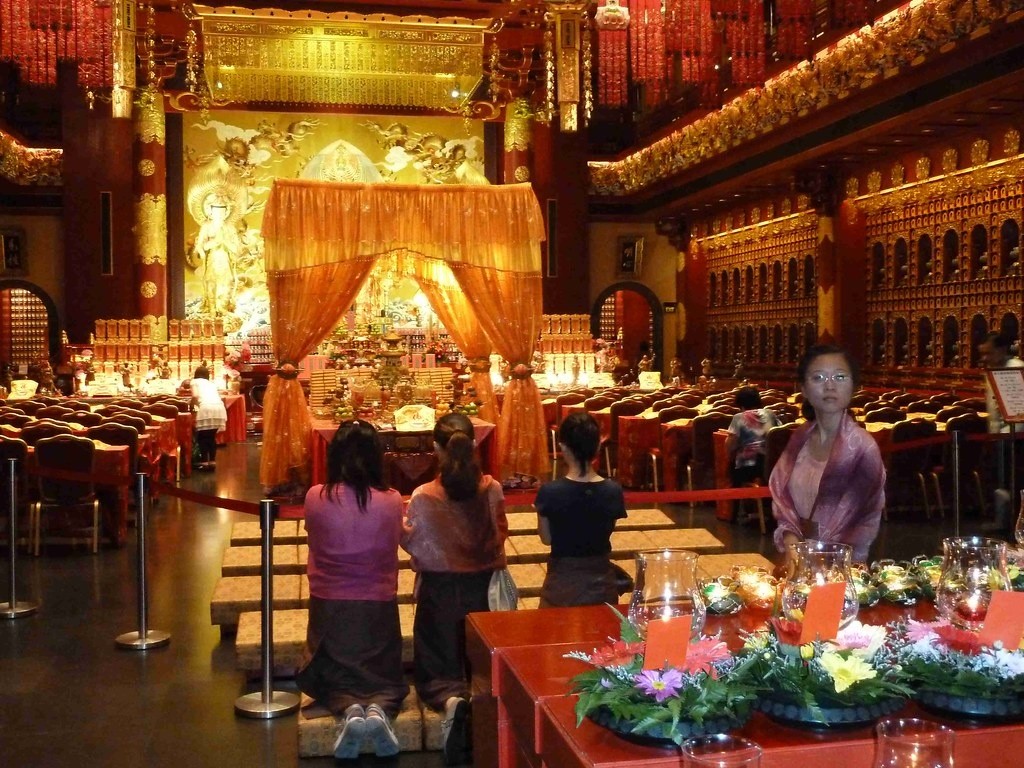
[808,372,853,383]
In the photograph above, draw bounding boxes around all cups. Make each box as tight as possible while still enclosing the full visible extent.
[629,551,706,642]
[1015,490,1024,550]
[783,541,859,630]
[937,537,1013,633]
[874,719,956,768]
[682,734,761,768]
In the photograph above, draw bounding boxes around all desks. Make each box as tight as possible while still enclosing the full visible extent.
[464,585,1024,768]
[310,412,496,485]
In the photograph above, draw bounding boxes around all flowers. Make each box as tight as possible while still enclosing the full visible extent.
[562,602,1024,746]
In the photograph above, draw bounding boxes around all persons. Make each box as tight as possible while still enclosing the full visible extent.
[977,330,1024,534]
[768,342,886,570]
[294,415,411,760]
[400,411,520,766]
[187,366,228,472]
[533,412,633,608]
[724,385,783,531]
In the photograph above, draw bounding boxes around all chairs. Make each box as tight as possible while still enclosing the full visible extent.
[0,394,189,556]
[543,387,1024,535]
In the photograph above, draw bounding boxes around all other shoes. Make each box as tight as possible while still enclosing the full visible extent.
[196,464,216,472]
[440,696,474,767]
[737,512,758,524]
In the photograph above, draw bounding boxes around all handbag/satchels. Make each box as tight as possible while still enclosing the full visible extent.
[488,567,518,614]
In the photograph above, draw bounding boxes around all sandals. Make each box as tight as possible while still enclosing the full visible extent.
[365,703,401,756]
[332,703,365,757]
